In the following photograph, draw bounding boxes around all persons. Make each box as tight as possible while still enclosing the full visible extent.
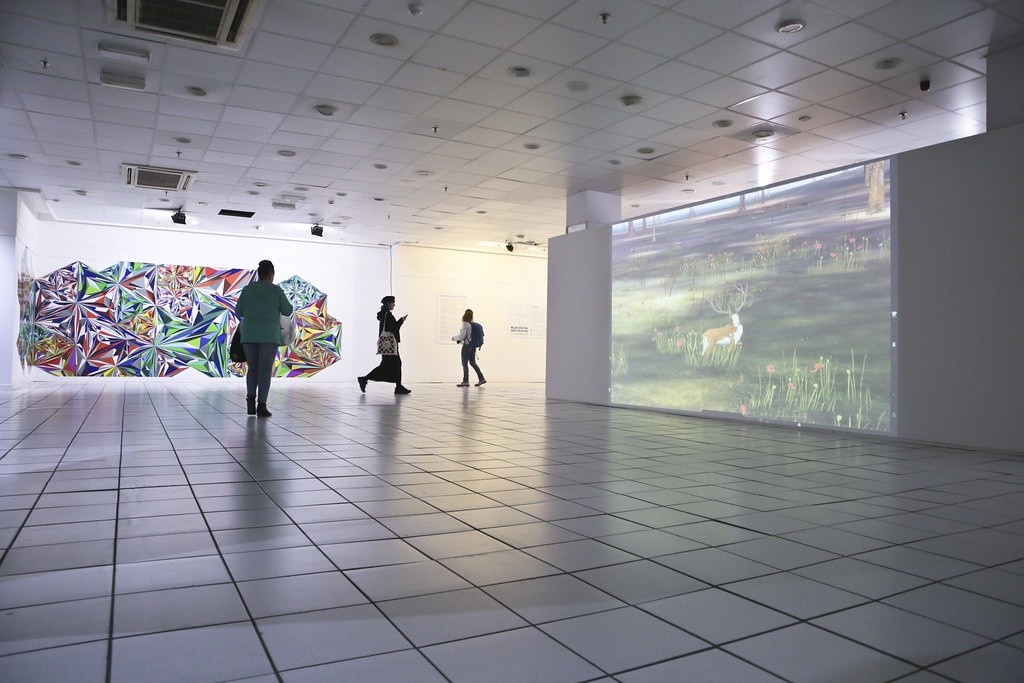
[451,309,487,387]
[357,296,412,395]
[234,261,294,418]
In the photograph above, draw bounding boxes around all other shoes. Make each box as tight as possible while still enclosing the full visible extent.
[257,403,272,417]
[358,377,367,393]
[457,382,470,387]
[475,378,488,386]
[246,397,256,415]
[394,385,411,394]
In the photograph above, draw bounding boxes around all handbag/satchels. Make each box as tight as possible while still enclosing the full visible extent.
[230,323,248,362]
[279,316,297,347]
[376,311,397,355]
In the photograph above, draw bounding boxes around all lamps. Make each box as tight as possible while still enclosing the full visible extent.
[506,243,514,252]
[311,223,324,237]
[171,210,186,224]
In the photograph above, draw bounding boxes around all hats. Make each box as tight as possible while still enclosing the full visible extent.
[381,296,395,303]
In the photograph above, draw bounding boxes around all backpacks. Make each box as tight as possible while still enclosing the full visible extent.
[465,321,484,348]
